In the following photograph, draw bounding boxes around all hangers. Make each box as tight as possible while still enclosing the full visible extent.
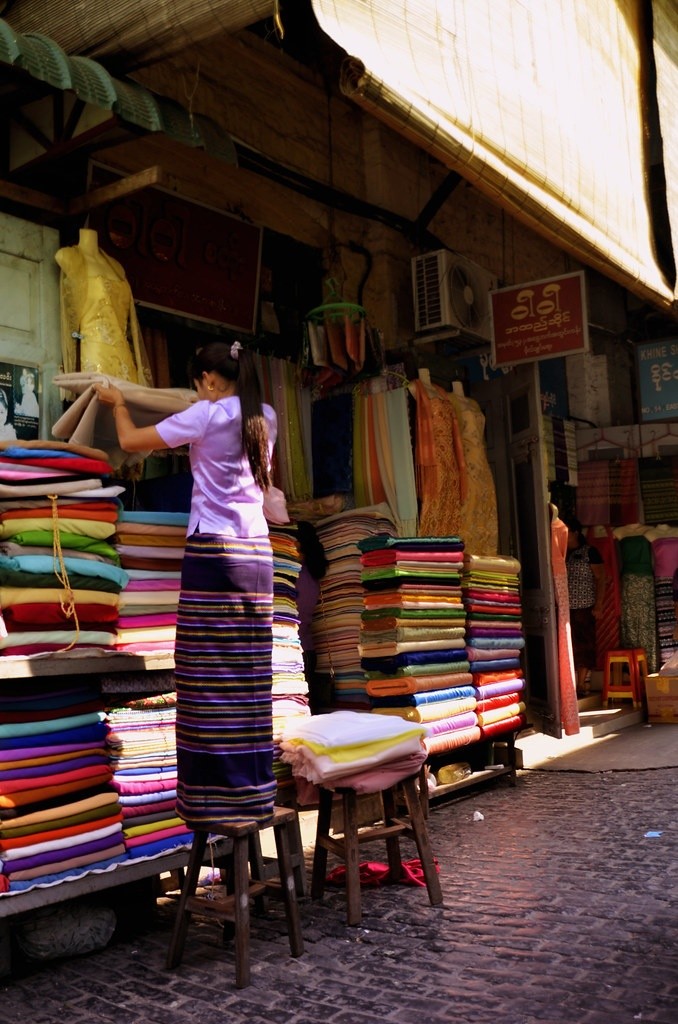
[352,365,413,402]
[572,418,678,463]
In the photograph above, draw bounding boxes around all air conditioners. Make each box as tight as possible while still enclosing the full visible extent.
[411,248,498,354]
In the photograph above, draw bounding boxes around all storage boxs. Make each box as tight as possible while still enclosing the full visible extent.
[644,673,678,723]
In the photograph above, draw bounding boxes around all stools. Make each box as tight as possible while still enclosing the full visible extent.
[311,770,443,926]
[166,805,304,990]
[602,647,651,709]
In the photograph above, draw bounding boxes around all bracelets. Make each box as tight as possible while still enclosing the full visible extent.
[113,403,127,417]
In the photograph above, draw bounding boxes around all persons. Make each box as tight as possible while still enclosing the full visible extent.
[644,524,678,667]
[614,522,656,677]
[566,514,604,697]
[56,228,147,389]
[408,368,459,535]
[93,343,277,817]
[446,382,497,555]
[550,506,579,734]
